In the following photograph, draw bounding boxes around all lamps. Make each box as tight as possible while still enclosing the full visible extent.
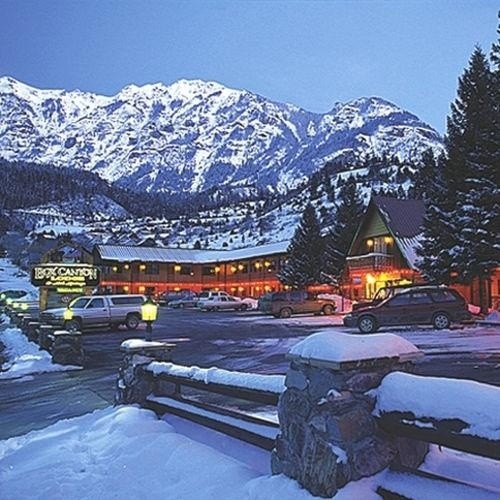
[63,305,73,332]
[140,294,158,343]
[0,293,28,313]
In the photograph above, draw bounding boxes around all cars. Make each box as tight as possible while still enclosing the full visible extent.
[344,285,472,334]
[168,291,248,312]
[39,294,147,331]
[0,289,32,306]
[258,291,337,318]
